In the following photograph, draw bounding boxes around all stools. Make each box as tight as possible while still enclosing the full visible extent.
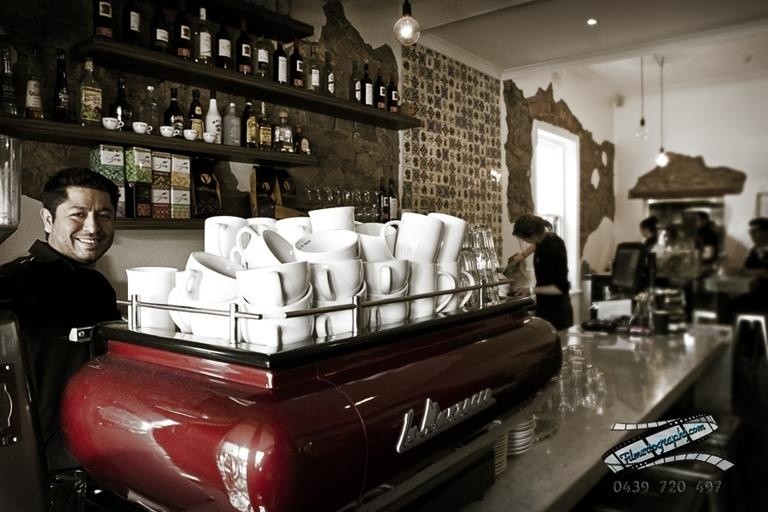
[638,411,743,512]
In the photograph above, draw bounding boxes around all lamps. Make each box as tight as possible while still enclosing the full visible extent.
[390,0,420,49]
[635,57,650,145]
[653,55,671,167]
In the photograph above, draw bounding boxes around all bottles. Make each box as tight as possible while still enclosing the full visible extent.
[291,37,305,87]
[53,52,72,123]
[214,10,233,69]
[152,2,172,52]
[274,32,288,84]
[175,1,193,56]
[78,51,104,125]
[255,25,271,77]
[196,7,213,64]
[235,16,253,74]
[23,73,45,122]
[108,77,298,151]
[122,3,142,45]
[379,175,399,220]
[324,48,336,95]
[2,51,18,120]
[95,0,117,40]
[349,54,399,113]
[310,42,322,90]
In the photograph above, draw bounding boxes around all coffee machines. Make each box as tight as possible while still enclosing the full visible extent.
[56,293,561,511]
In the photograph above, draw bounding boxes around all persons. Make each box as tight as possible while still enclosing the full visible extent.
[641,211,718,313]
[745,217,768,314]
[512,214,573,332]
[0,168,121,452]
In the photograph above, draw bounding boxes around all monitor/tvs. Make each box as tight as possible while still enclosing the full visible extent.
[609,242,648,294]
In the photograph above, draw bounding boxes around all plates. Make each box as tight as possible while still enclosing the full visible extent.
[488,415,540,479]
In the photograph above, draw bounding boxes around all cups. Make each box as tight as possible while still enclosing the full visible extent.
[183,129,201,140]
[539,339,603,413]
[503,253,530,288]
[203,132,220,142]
[159,126,179,137]
[461,225,502,308]
[101,117,125,130]
[132,122,153,134]
[123,205,475,343]
[653,311,670,335]
[294,184,380,221]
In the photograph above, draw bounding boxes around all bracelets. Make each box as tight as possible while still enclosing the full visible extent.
[520,252,527,258]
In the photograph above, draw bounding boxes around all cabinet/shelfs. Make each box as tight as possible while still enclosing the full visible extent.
[0,0,420,234]
